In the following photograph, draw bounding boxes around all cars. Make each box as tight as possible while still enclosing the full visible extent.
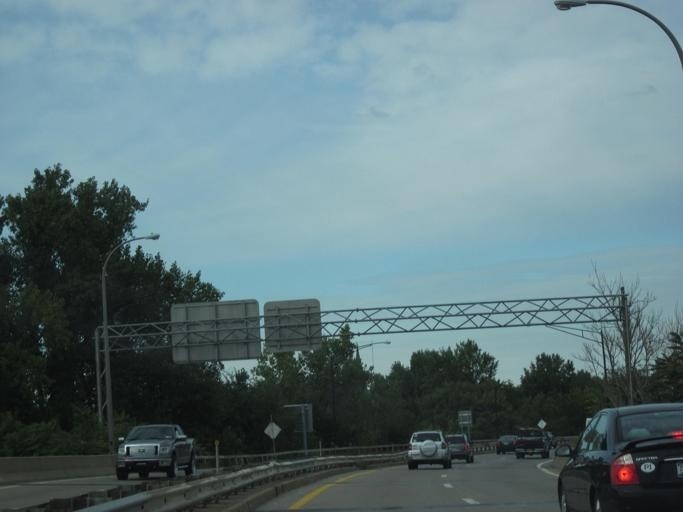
[553,400,682,512]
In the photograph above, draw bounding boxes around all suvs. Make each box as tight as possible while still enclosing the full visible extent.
[111,421,199,482]
[404,426,559,471]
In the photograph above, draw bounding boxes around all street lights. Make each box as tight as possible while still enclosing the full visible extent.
[97,230,162,455]
[329,340,391,455]
